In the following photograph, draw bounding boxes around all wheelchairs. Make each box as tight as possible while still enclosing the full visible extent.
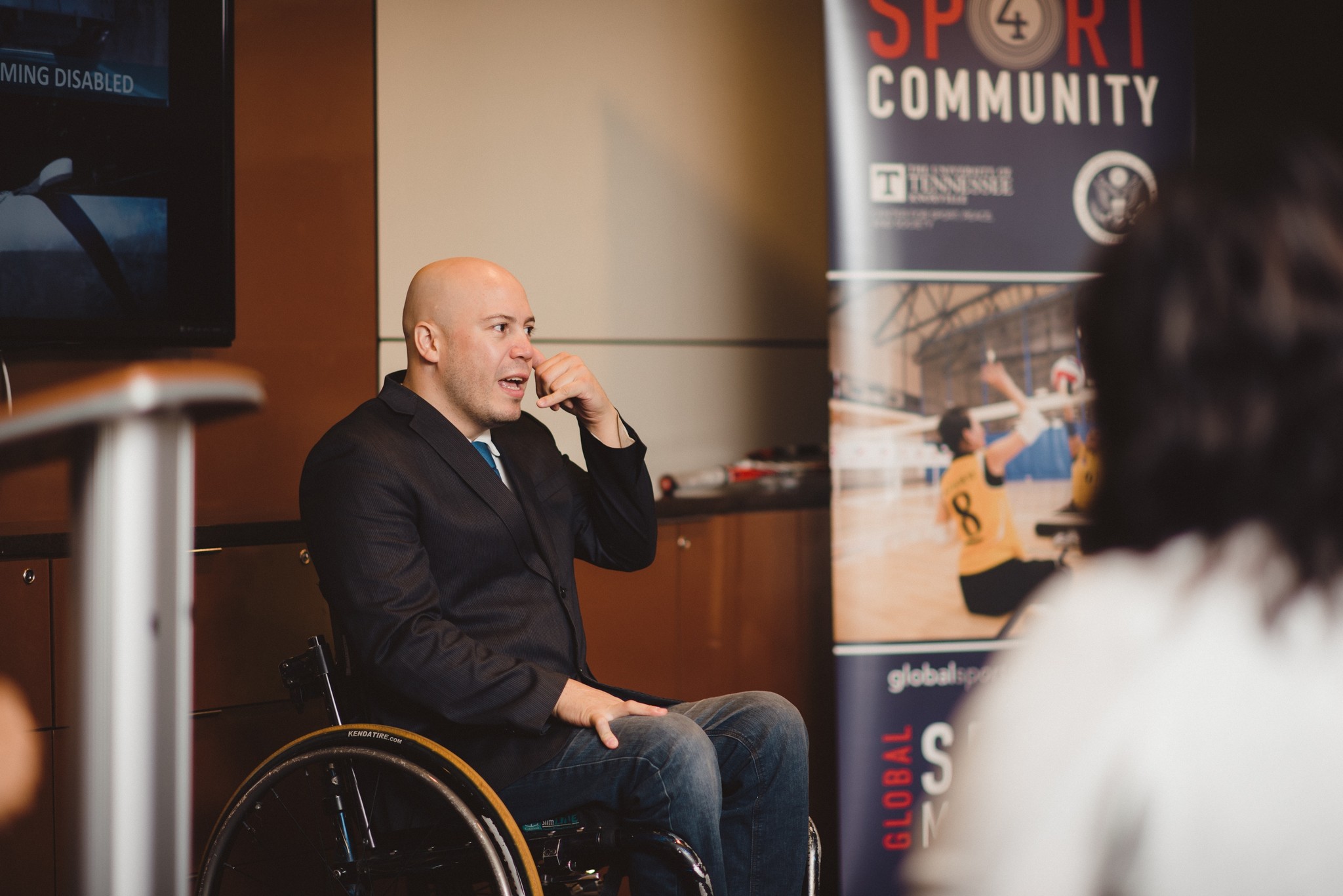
[194,629,824,895]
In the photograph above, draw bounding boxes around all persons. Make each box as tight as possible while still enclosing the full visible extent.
[301,257,807,896]
[900,0,1343,896]
[1036,376,1102,556]
[933,361,1061,615]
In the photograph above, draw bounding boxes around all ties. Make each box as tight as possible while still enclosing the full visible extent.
[471,441,502,481]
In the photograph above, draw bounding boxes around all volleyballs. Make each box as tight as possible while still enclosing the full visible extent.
[1050,354,1085,395]
